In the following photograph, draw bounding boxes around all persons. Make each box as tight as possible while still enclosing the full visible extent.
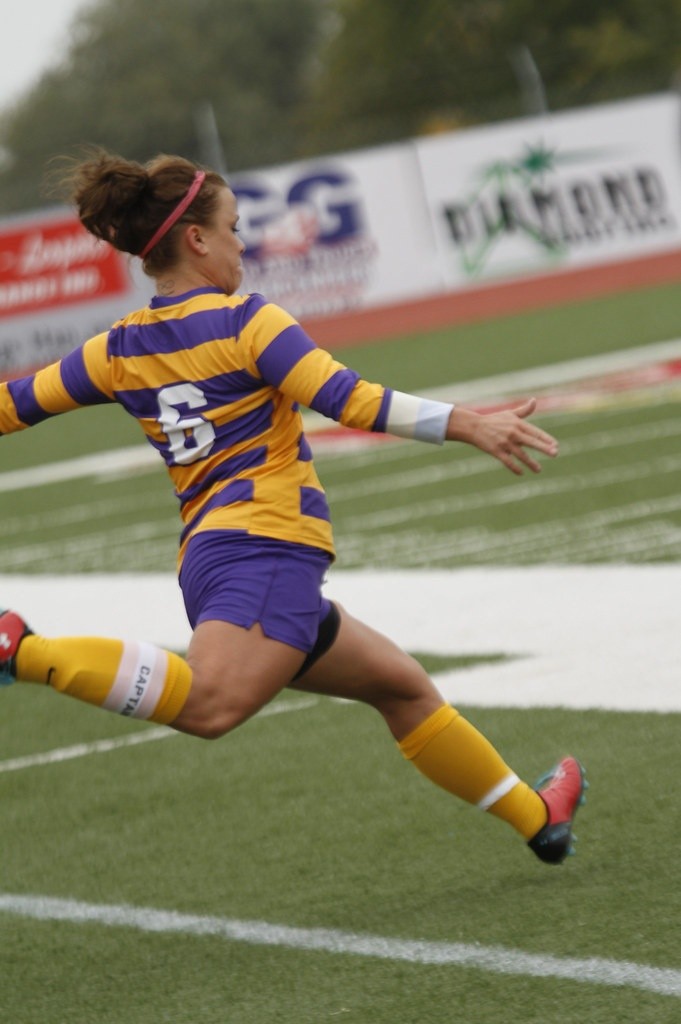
[0,145,589,864]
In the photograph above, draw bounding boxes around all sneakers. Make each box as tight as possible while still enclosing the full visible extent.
[0,608,35,684]
[527,755,589,865]
